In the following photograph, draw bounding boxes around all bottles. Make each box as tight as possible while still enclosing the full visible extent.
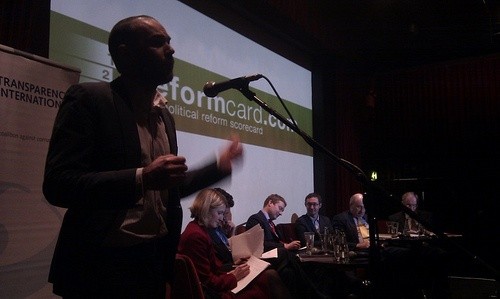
[320,225,349,262]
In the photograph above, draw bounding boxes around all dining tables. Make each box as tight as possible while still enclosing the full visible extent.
[298,245,376,297]
[379,240,448,299]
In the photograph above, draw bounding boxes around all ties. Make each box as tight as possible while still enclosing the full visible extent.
[314,220,320,234]
[357,219,369,240]
[269,221,279,240]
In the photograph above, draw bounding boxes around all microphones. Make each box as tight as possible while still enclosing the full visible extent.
[203,73,262,97]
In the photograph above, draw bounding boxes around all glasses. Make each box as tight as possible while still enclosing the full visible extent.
[405,203,418,209]
[305,202,320,206]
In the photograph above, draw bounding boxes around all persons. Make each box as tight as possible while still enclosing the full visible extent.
[390,192,447,299]
[246,194,301,252]
[177,189,290,299]
[331,193,371,250]
[294,193,372,299]
[208,187,330,299]
[42,14,246,299]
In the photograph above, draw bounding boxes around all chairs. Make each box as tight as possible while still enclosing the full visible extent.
[173,220,408,299]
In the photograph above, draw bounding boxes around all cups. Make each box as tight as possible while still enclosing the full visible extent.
[386,221,399,238]
[304,232,314,255]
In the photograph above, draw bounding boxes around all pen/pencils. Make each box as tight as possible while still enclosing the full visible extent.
[232,264,240,268]
